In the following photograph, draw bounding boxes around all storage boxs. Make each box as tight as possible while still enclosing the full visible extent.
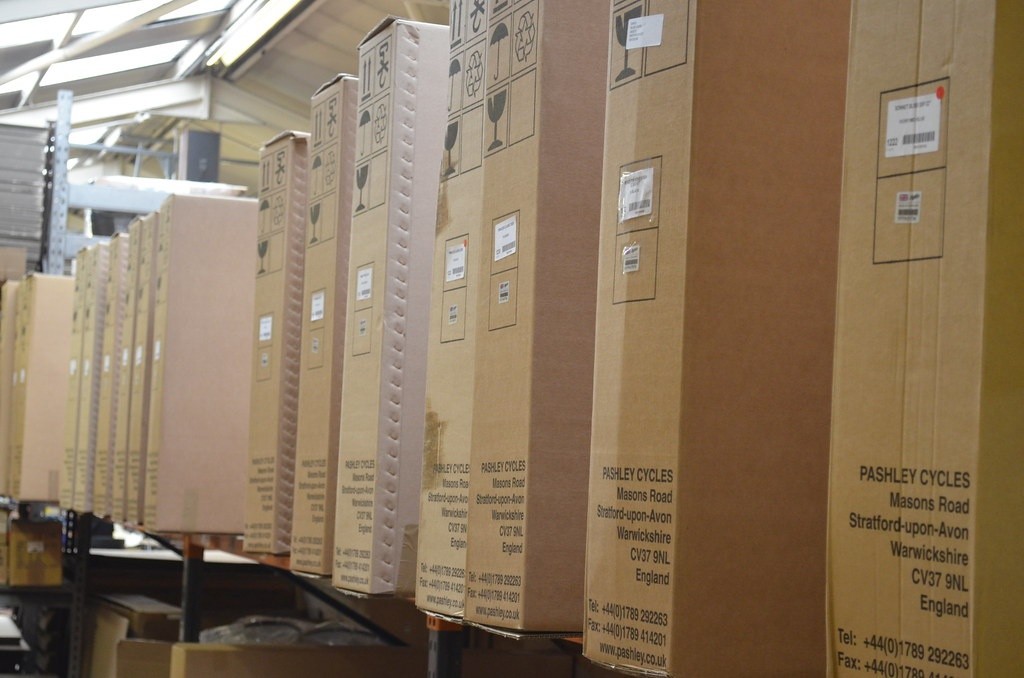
[0,0,1024,678]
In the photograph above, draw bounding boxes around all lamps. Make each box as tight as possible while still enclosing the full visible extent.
[205,0,306,69]
